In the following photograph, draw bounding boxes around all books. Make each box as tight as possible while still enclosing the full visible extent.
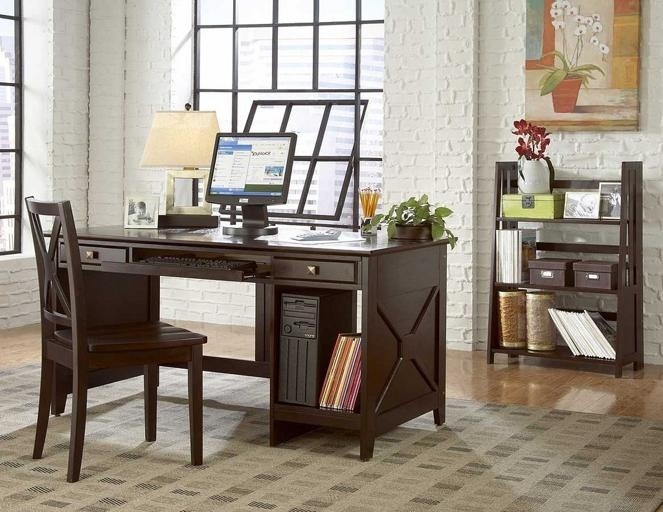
[496,229,536,284]
[548,308,617,359]
[318,333,362,412]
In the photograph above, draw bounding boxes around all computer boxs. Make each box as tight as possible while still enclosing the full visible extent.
[277,289,352,407]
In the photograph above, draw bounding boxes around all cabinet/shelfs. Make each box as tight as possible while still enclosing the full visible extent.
[487,161,643,378]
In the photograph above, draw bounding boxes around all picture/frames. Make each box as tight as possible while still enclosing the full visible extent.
[563,191,601,220]
[598,182,628,220]
[124,196,159,229]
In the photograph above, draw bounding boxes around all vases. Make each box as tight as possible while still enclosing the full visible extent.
[551,78,582,113]
[516,160,551,195]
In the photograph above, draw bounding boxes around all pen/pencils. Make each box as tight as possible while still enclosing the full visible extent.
[359,187,381,217]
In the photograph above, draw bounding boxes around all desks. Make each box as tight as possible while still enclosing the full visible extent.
[39,224,458,460]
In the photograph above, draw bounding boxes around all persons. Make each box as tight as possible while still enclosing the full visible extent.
[608,185,621,216]
[565,193,597,217]
[128,202,153,225]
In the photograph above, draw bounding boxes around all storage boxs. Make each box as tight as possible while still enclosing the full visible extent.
[501,193,565,219]
[573,260,627,290]
[527,257,582,286]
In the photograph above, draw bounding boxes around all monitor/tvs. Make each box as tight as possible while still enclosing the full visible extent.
[204,133,296,236]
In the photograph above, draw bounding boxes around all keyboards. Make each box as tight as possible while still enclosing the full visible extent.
[138,254,256,272]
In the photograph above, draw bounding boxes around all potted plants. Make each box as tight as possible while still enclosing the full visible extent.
[360,193,457,250]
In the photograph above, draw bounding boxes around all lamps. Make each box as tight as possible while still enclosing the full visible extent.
[139,101,220,227]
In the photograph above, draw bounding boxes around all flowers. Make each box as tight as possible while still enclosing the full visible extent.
[512,120,554,161]
[534,1,610,97]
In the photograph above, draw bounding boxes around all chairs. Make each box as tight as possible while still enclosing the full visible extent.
[22,195,207,483]
